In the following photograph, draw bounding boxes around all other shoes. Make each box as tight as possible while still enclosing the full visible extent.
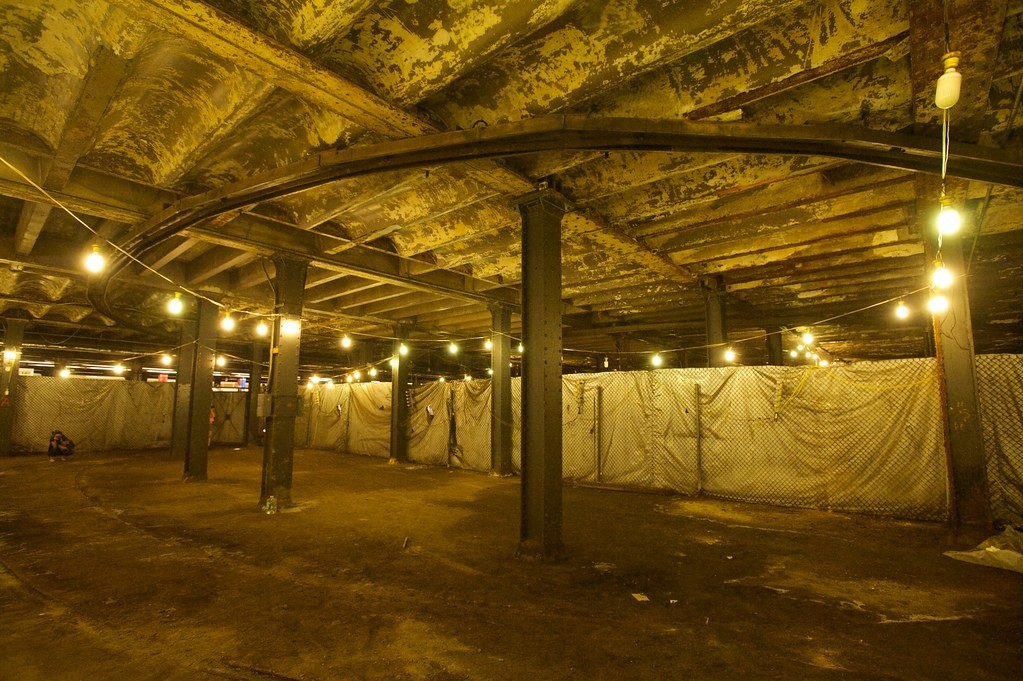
[60,457,65,461]
[50,458,55,463]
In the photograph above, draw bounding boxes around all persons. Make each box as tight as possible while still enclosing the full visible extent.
[48,430,75,462]
[208,403,215,450]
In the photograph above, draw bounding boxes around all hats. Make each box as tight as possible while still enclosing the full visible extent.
[52,430,62,437]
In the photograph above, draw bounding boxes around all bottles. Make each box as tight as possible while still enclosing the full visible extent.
[266,496,277,514]
